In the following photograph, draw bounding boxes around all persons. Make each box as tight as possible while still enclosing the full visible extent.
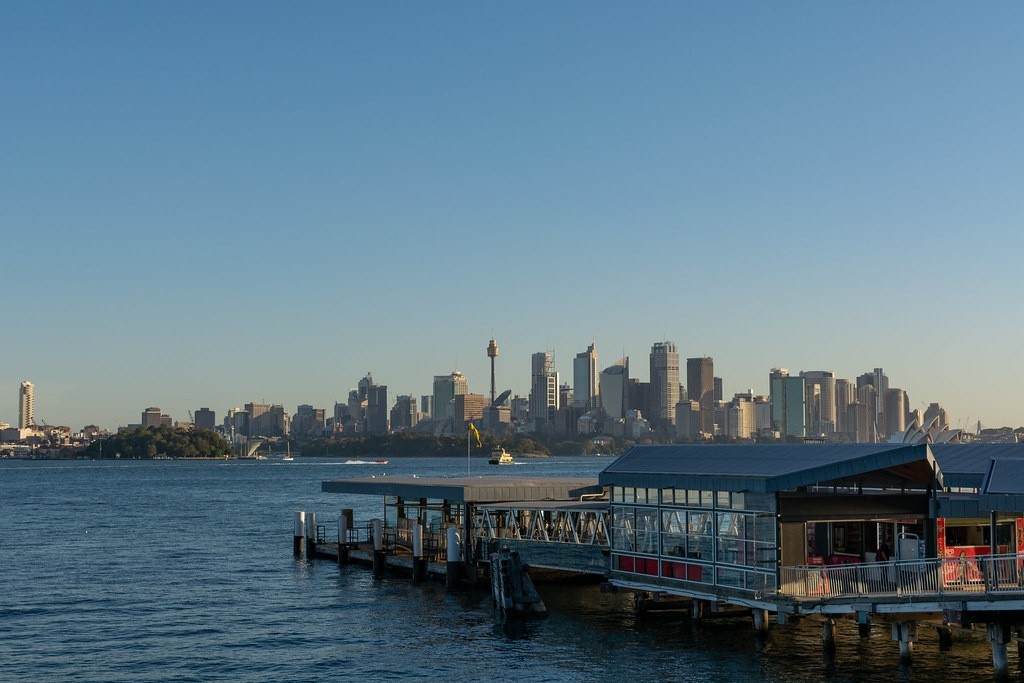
[476,519,484,536]
[876,539,891,589]
[514,519,561,540]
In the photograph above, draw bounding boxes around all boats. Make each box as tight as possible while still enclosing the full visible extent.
[375,461,388,465]
[488,445,513,465]
[283,458,294,461]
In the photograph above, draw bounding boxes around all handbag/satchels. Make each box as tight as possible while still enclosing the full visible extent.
[874,544,889,561]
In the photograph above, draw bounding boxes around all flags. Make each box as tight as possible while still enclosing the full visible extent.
[470,423,481,447]
